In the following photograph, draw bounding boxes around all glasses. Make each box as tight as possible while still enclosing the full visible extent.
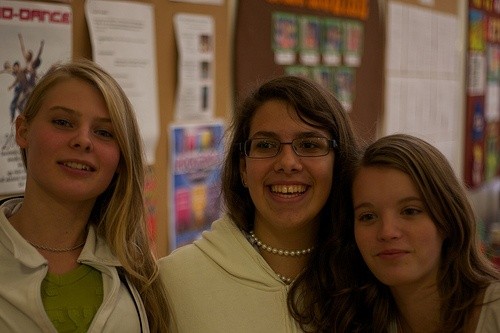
[240,135,337,158]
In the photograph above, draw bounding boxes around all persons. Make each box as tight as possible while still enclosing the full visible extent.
[157,76,358,333]
[352,134,500,333]
[0,59,178,333]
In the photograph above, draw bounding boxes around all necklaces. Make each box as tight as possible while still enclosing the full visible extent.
[28,235,87,256]
[248,226,317,283]
